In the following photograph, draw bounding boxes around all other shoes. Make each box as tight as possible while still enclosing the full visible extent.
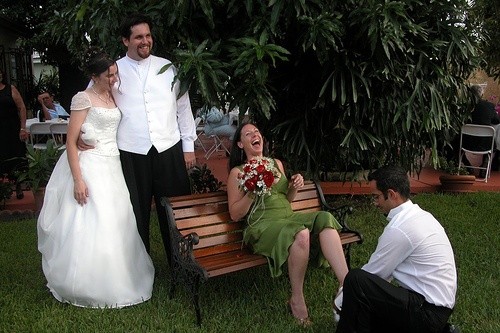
[288,300,309,327]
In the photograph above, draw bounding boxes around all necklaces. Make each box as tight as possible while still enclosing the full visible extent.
[91,86,109,105]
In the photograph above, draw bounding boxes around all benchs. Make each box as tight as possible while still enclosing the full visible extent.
[161,179,364,326]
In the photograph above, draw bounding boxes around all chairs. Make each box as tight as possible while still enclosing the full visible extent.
[194,109,238,161]
[458,124,497,183]
[26,110,68,150]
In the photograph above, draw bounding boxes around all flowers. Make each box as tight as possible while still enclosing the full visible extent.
[237,155,281,199]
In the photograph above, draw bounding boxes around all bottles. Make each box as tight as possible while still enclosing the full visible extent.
[39,109,45,122]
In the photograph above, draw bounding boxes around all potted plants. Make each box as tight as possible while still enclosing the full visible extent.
[439,157,475,192]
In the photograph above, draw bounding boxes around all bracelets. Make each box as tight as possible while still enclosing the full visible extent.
[20,128,26,131]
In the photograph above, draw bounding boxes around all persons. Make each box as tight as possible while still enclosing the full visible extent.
[227,123,349,328]
[203,105,237,157]
[37,48,155,309]
[38,92,70,120]
[436,85,500,178]
[76,16,198,268]
[332,165,457,333]
[0,72,28,200]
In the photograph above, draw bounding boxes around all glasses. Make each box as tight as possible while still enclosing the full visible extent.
[371,192,387,204]
